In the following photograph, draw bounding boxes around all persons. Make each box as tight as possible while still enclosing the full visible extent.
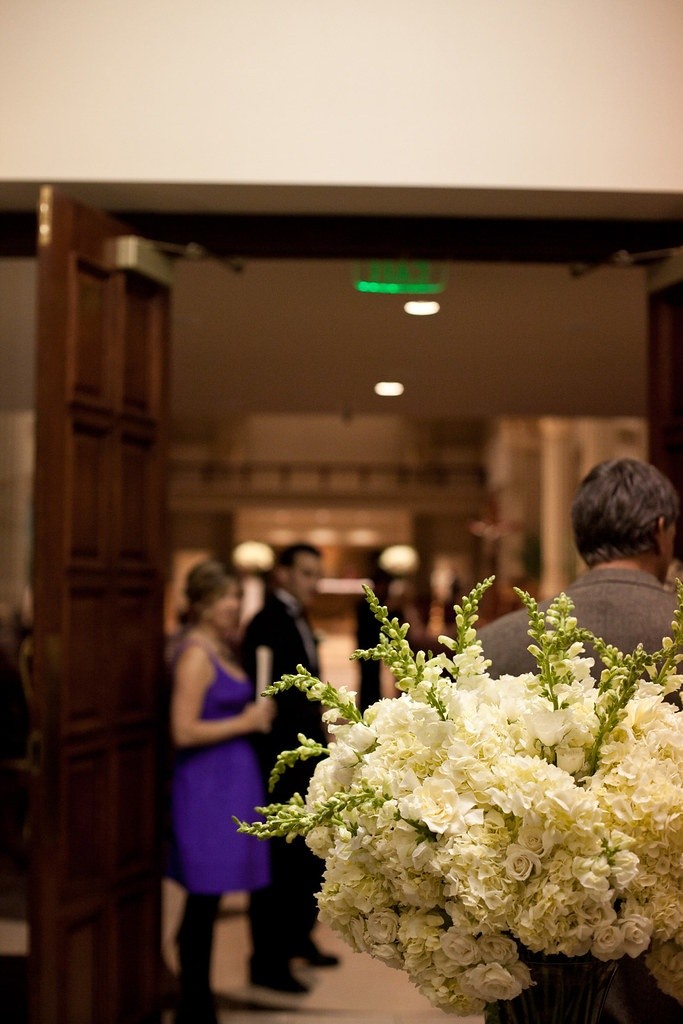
[167,543,461,1024]
[477,455,683,1024]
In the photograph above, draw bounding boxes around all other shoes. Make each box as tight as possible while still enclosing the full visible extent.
[250,973,308,993]
[288,936,338,966]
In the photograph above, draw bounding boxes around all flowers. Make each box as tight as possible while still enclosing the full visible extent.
[231,570,682,1015]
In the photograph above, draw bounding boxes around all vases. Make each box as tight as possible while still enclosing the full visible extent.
[487,931,630,1024]
